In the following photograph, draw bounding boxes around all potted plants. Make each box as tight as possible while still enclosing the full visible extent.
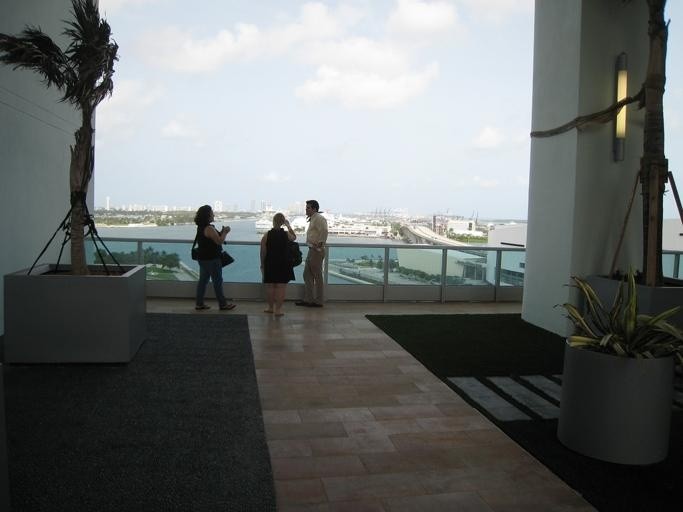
[553,262,683,466]
[0,0,146,364]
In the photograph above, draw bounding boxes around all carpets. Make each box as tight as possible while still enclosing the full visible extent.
[0,313,277,512]
[365,313,683,512]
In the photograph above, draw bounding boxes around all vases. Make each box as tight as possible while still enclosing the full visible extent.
[583,275,683,331]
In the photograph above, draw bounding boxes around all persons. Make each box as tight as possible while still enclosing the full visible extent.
[295,199,329,306]
[195,204,236,310]
[260,213,297,316]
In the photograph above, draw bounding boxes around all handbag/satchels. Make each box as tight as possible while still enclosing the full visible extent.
[288,241,302,267]
[192,229,200,260]
[222,251,234,267]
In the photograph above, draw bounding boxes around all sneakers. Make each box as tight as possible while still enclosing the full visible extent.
[196,305,211,310]
[296,302,323,307]
[220,303,235,310]
